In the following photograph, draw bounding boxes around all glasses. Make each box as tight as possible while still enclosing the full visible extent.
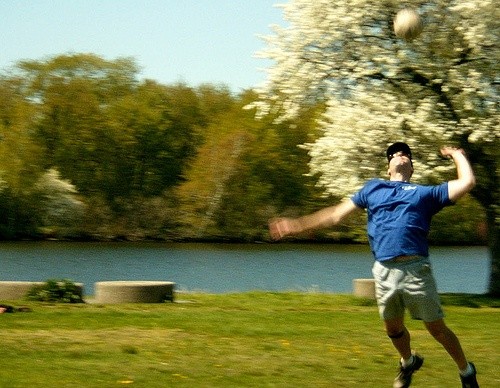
[389,153,411,161]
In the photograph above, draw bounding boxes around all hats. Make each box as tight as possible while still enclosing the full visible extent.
[386,141,412,162]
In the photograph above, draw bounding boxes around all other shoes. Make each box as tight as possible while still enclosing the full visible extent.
[459,361,479,388]
[392,350,424,388]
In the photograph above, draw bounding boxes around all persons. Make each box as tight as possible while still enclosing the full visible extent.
[268,142,480,388]
